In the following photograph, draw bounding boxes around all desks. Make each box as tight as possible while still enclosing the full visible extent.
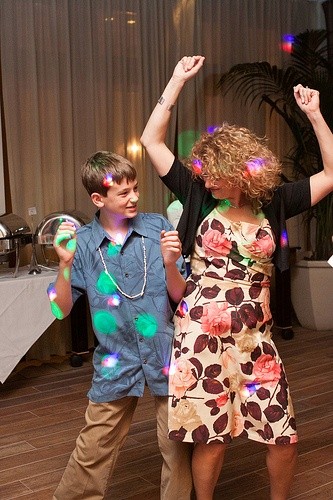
[0,266,92,386]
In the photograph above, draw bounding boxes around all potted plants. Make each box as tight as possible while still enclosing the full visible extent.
[219,25,333,331]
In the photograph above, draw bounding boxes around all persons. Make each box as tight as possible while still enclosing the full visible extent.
[46,150,193,500]
[140,56,333,500]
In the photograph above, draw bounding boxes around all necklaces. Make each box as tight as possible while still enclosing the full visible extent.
[98,237,147,299]
[224,200,244,208]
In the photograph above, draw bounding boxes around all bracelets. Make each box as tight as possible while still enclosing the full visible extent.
[158,97,175,111]
